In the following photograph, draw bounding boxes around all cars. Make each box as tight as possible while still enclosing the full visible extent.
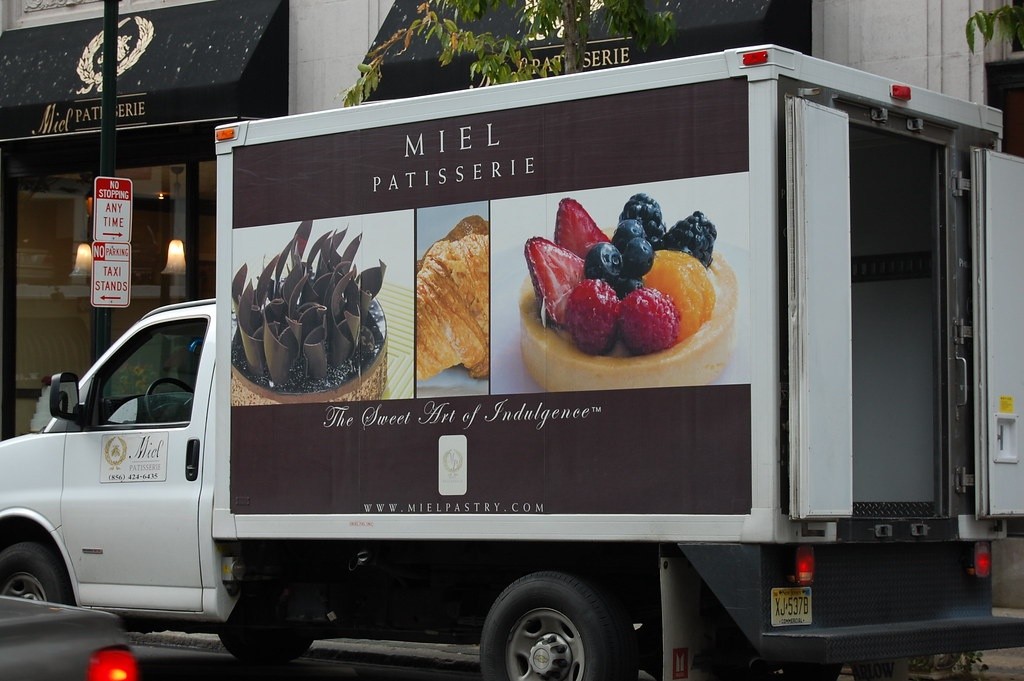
[0,595,137,681]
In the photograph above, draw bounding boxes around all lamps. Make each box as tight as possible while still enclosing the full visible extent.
[161,167,186,276]
[68,169,93,278]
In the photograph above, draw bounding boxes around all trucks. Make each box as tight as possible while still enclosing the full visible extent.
[0,46,1023,681]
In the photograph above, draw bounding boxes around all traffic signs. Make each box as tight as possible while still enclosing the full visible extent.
[91,241,132,309]
[93,177,133,243]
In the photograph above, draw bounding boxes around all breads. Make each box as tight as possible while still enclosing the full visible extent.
[415,214,490,378]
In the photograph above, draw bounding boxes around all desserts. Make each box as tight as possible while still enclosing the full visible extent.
[517,192,736,387]
[228,218,387,405]
